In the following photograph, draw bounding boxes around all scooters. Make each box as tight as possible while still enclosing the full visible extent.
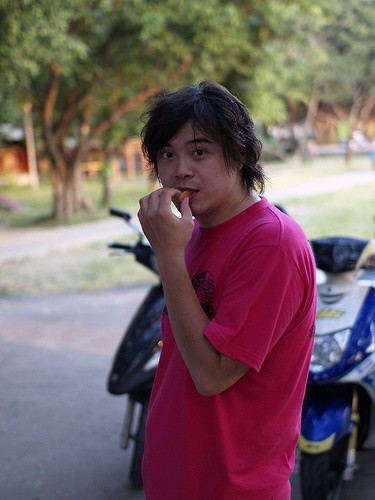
[107,206,168,485]
[297,236,375,500]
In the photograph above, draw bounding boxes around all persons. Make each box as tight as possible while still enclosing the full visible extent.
[135,81,318,498]
